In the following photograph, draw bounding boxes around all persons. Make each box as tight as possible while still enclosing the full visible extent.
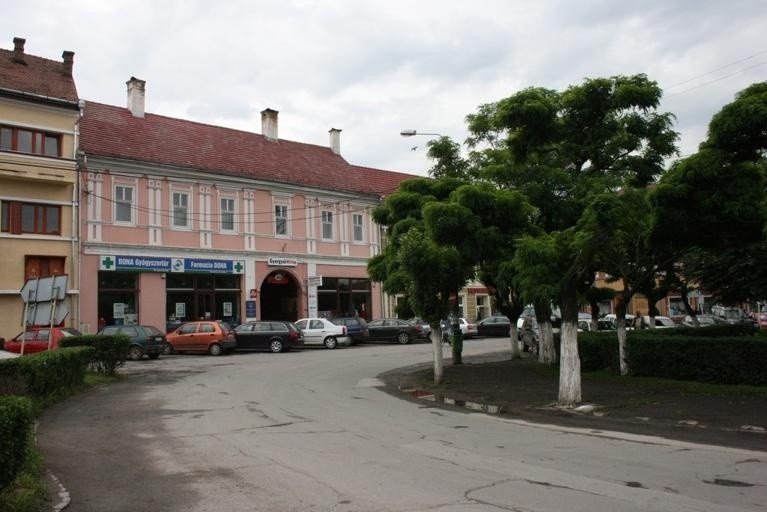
[694,306,698,314]
[630,310,645,330]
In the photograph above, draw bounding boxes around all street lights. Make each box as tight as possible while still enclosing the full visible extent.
[399,129,444,139]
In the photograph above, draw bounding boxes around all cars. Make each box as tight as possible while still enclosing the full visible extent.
[328,316,371,345]
[516,303,767,331]
[365,318,424,345]
[521,315,563,356]
[231,318,306,355]
[457,316,479,337]
[0,349,21,359]
[410,317,449,344]
[293,317,352,351]
[161,319,238,358]
[475,316,510,339]
[95,322,169,363]
[1,325,86,354]
